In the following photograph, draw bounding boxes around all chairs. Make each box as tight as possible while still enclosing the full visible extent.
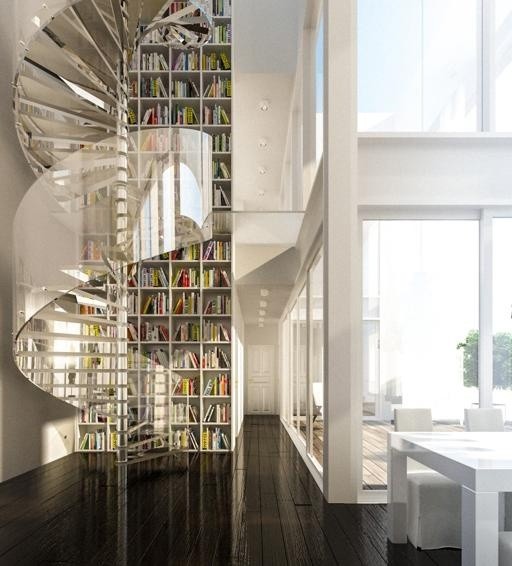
[463,408,512,530]
[498,531,512,566]
[313,382,324,426]
[393,408,462,550]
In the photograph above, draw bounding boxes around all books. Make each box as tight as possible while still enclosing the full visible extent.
[171,243,200,260]
[141,349,169,369]
[140,76,168,97]
[171,78,200,98]
[127,321,138,341]
[202,373,231,396]
[172,292,201,315]
[142,292,169,314]
[213,184,230,206]
[213,211,231,234]
[202,318,230,341]
[202,241,231,260]
[127,48,136,71]
[110,428,117,450]
[172,321,200,342]
[212,0,232,17]
[203,103,229,125]
[127,269,138,287]
[174,50,200,71]
[127,291,138,315]
[162,0,200,18]
[202,52,231,71]
[141,52,168,71]
[172,348,200,369]
[211,133,231,152]
[143,252,169,260]
[82,322,117,339]
[127,107,137,125]
[80,305,107,314]
[174,376,200,396]
[84,357,103,369]
[127,345,138,370]
[140,321,169,342]
[202,427,231,450]
[172,102,199,125]
[208,24,231,43]
[212,158,231,179]
[173,427,199,450]
[129,434,168,449]
[171,267,200,288]
[203,74,231,98]
[80,427,107,450]
[202,267,231,287]
[140,267,169,287]
[202,345,230,369]
[141,102,168,125]
[172,403,199,423]
[83,343,102,353]
[202,402,231,423]
[140,23,200,44]
[128,80,138,98]
[80,274,107,287]
[204,294,231,314]
[81,402,113,423]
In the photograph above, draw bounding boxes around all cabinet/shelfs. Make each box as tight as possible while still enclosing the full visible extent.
[73,0,236,452]
[75,453,235,556]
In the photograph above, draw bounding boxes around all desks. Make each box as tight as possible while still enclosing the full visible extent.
[386,432,512,566]
